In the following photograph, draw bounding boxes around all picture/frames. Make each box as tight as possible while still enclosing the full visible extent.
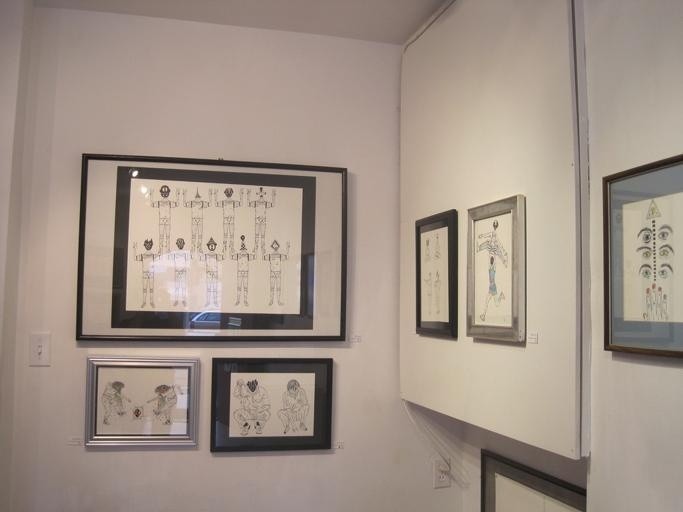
[72,150,352,343]
[415,197,524,344]
[479,447,587,510]
[82,356,334,455]
[603,146,683,357]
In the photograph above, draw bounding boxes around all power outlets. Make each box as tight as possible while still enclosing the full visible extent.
[433,458,451,488]
